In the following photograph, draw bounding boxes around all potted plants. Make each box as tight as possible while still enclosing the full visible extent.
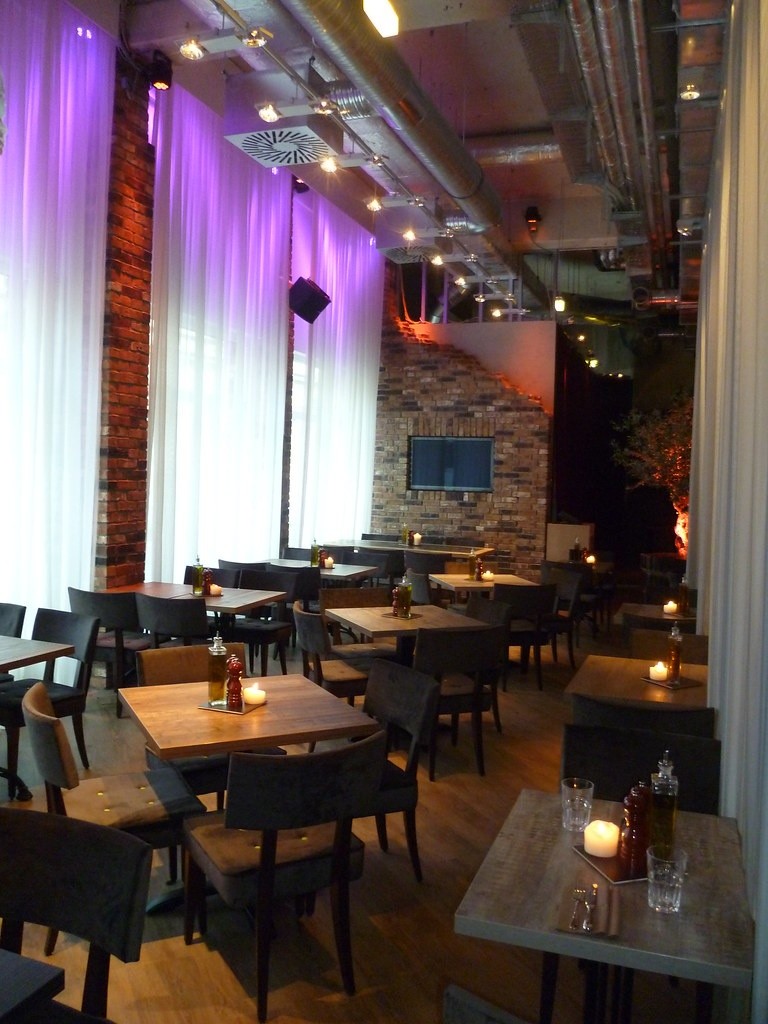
[612,387,694,554]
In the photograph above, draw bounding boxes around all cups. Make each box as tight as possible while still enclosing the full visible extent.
[646,843,689,913]
[560,777,595,833]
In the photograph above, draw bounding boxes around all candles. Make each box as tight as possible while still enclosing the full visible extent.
[649,661,667,681]
[210,584,222,594]
[584,820,620,858]
[482,570,494,580]
[663,600,677,614]
[243,683,267,705]
[325,557,333,568]
[414,532,421,545]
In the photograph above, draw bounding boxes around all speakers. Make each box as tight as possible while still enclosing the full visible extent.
[288,277,331,325]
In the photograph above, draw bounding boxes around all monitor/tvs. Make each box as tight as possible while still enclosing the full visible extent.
[406,435,496,493]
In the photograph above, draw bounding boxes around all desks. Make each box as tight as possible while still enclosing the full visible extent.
[619,602,697,625]
[427,574,541,611]
[562,653,709,712]
[454,788,754,1023]
[318,539,495,576]
[266,558,380,643]
[118,673,382,939]
[108,580,288,644]
[323,604,489,753]
[0,634,75,800]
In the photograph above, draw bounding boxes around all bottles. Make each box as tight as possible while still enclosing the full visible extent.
[677,577,690,616]
[401,524,408,545]
[651,757,679,833]
[310,540,319,567]
[207,637,226,706]
[667,627,681,679]
[468,550,476,580]
[193,559,202,593]
[399,575,412,616]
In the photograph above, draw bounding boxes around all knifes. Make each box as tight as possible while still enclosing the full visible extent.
[581,883,598,934]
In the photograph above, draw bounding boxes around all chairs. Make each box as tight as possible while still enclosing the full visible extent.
[0,532,723,1024]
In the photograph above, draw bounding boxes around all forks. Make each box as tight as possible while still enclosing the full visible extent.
[568,888,585,933]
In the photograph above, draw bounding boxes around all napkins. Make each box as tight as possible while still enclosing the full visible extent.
[555,883,621,940]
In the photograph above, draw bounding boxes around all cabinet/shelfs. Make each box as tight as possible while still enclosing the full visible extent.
[546,522,594,562]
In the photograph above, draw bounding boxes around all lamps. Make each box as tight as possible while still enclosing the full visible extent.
[148,25,526,325]
[679,83,701,100]
